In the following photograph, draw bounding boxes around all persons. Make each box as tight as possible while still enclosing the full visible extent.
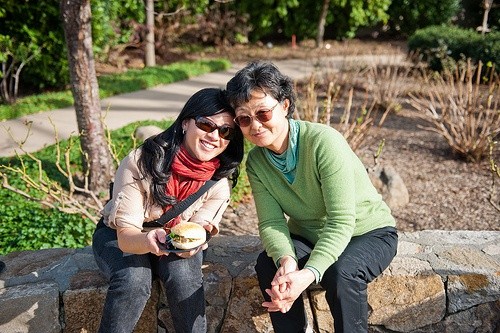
[228,60,399,333]
[92,88,245,333]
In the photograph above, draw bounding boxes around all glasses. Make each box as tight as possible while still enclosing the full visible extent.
[234,101,279,127]
[192,116,236,140]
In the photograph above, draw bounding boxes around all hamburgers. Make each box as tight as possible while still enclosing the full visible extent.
[169,222,206,250]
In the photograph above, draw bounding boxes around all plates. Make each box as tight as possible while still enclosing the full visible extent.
[159,229,211,252]
